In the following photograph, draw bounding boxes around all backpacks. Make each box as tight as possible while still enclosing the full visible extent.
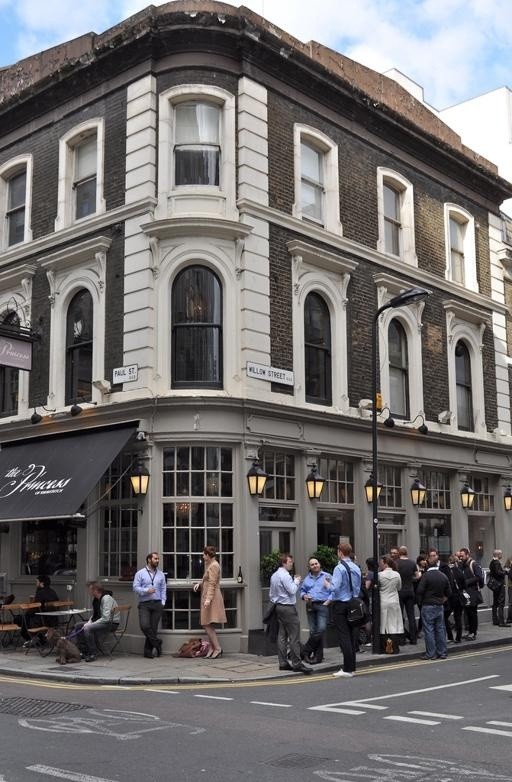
[470,559,484,588]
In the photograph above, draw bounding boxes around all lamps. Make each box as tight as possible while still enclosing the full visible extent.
[404,414,428,434]
[130,461,150,495]
[501,484,512,510]
[370,407,394,426]
[409,474,425,507]
[71,397,97,416]
[305,463,325,500]
[459,478,475,508]
[364,469,383,503]
[31,403,56,424]
[245,455,268,494]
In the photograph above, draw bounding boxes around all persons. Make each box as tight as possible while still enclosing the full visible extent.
[133,552,168,658]
[21,576,59,648]
[194,546,227,659]
[75,581,120,663]
[262,543,512,678]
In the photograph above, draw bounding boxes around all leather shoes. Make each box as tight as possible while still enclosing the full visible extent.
[420,654,446,660]
[144,640,162,658]
[499,623,511,626]
[279,650,322,672]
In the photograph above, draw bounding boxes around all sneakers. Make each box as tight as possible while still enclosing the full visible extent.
[333,669,353,677]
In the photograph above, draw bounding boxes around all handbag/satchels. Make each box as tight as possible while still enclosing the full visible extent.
[347,597,369,626]
[459,591,471,607]
[487,578,502,591]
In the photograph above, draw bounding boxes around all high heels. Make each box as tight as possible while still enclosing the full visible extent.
[203,650,223,659]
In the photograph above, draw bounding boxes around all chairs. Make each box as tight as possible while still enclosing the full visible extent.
[0,595,131,660]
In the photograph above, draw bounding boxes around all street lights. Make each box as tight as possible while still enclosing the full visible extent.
[365,284,432,654]
